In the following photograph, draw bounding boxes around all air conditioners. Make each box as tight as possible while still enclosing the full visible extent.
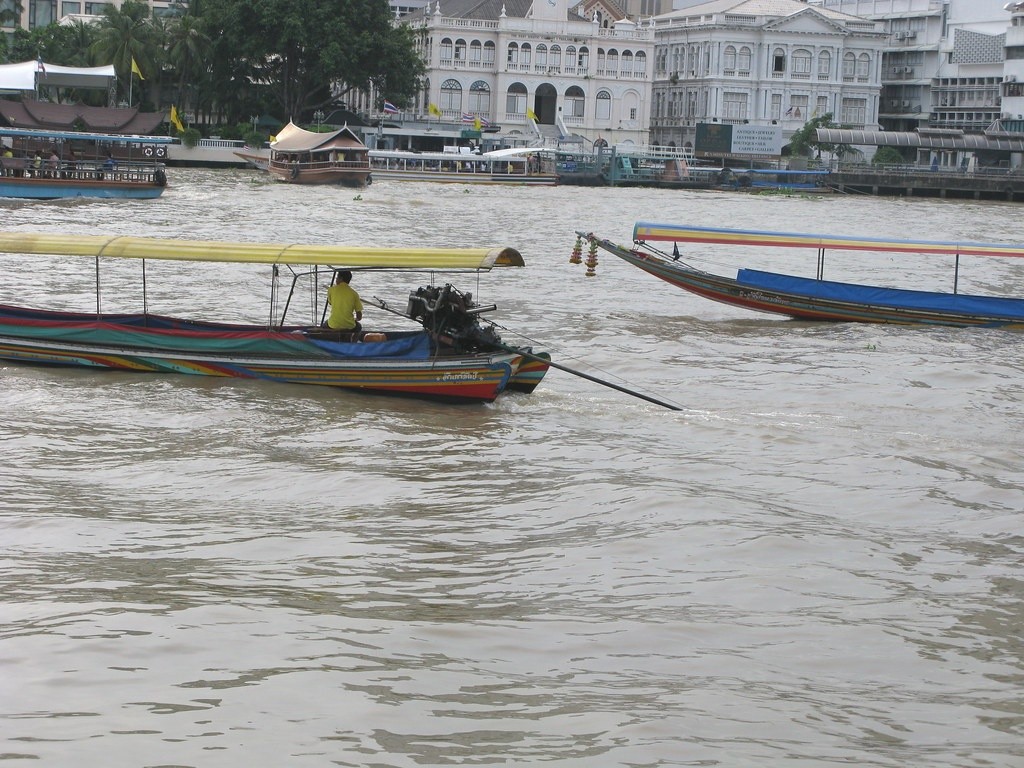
[1004,75,1017,82]
[902,101,910,107]
[895,32,906,41]
[1017,112,1023,119]
[906,30,918,39]
[890,100,898,107]
[892,67,901,73]
[903,66,912,73]
[1000,112,1010,119]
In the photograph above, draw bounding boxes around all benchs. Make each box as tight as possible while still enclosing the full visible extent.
[306,326,353,344]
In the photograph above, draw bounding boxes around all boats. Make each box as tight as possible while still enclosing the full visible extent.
[267,115,372,185]
[0,232,552,403]
[0,93,169,164]
[0,128,172,199]
[569,222,1024,333]
[232,148,559,183]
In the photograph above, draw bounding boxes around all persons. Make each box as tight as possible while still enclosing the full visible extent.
[456,151,543,177]
[278,146,364,168]
[0,145,118,183]
[36,91,130,109]
[321,270,362,344]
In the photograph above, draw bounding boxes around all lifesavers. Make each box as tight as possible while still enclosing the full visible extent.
[366,175,372,187]
[157,148,165,156]
[290,168,297,179]
[144,147,153,157]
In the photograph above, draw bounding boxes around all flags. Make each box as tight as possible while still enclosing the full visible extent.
[429,102,542,132]
[270,135,277,142]
[132,60,145,81]
[385,99,398,114]
[171,105,184,132]
[243,145,251,152]
